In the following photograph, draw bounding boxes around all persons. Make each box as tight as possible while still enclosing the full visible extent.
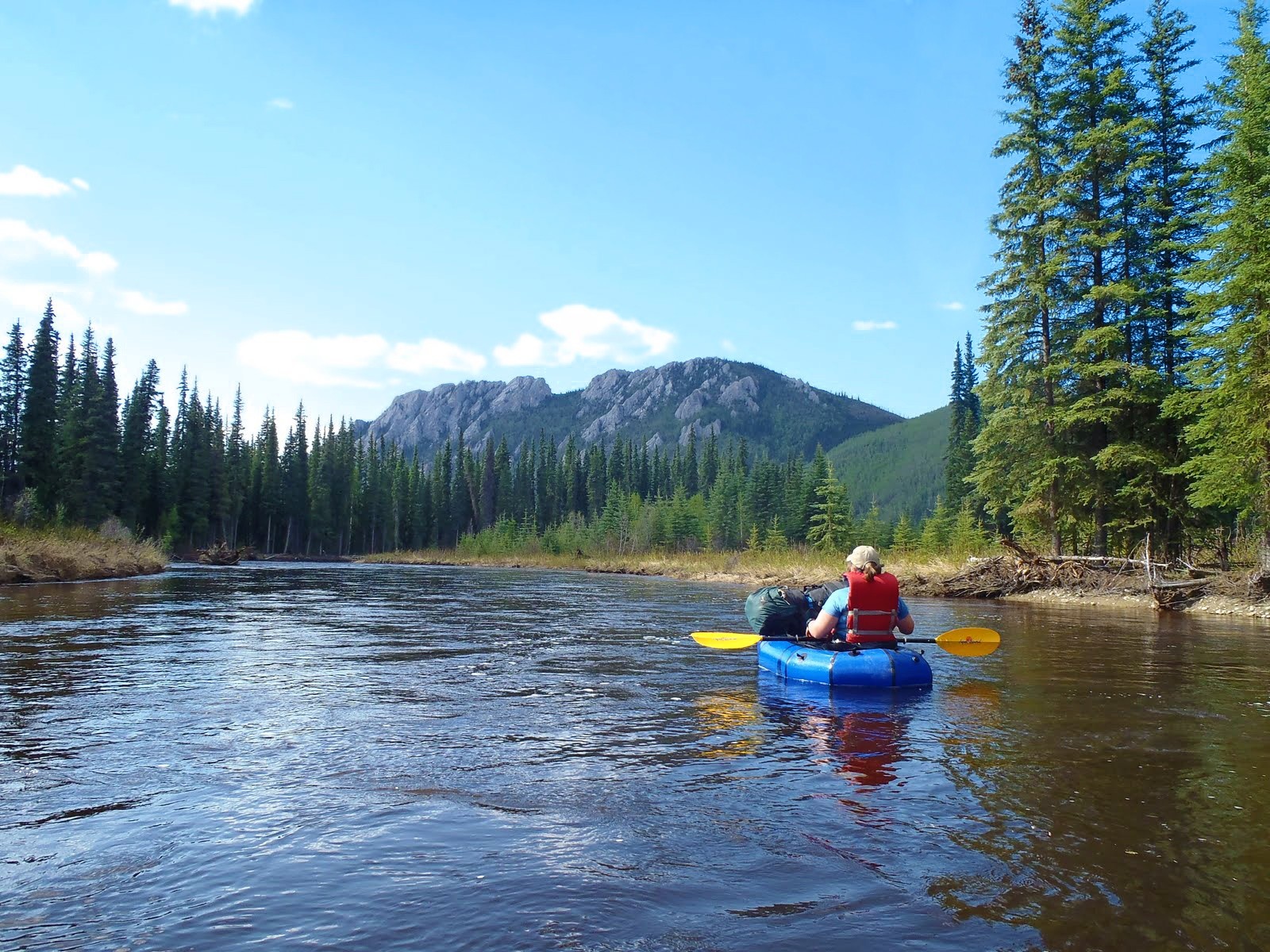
[805,545,914,645]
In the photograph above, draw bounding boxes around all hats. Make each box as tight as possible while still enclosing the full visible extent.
[846,545,880,569]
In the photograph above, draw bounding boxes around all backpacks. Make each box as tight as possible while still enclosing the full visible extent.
[745,580,851,636]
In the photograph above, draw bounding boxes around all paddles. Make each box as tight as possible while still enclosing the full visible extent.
[896,627,1001,656]
[690,631,828,650]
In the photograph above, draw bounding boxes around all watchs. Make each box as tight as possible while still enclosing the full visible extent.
[806,619,814,627]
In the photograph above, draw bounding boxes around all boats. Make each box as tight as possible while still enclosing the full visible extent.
[757,635,934,687]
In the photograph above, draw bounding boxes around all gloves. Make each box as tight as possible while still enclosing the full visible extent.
[803,608,819,627]
